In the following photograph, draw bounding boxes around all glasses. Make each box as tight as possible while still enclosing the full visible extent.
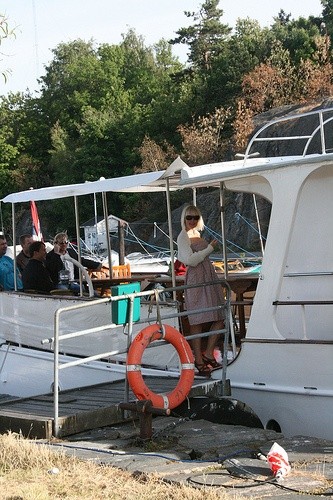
[184,215,200,220]
[55,241,68,245]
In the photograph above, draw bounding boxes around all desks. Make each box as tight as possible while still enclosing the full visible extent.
[148,273,261,337]
[69,274,161,297]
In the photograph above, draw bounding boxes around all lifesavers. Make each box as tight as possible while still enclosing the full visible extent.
[125,323,196,410]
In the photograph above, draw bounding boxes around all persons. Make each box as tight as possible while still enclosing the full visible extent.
[0,235,23,291]
[22,241,70,293]
[177,205,225,374]
[45,232,110,298]
[16,234,35,277]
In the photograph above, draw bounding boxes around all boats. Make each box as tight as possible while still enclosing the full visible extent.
[0,102,333,444]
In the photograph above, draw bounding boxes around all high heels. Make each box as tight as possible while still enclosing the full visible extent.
[194,361,212,376]
[202,354,222,368]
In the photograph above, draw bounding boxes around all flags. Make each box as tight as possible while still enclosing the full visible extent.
[28,187,43,241]
